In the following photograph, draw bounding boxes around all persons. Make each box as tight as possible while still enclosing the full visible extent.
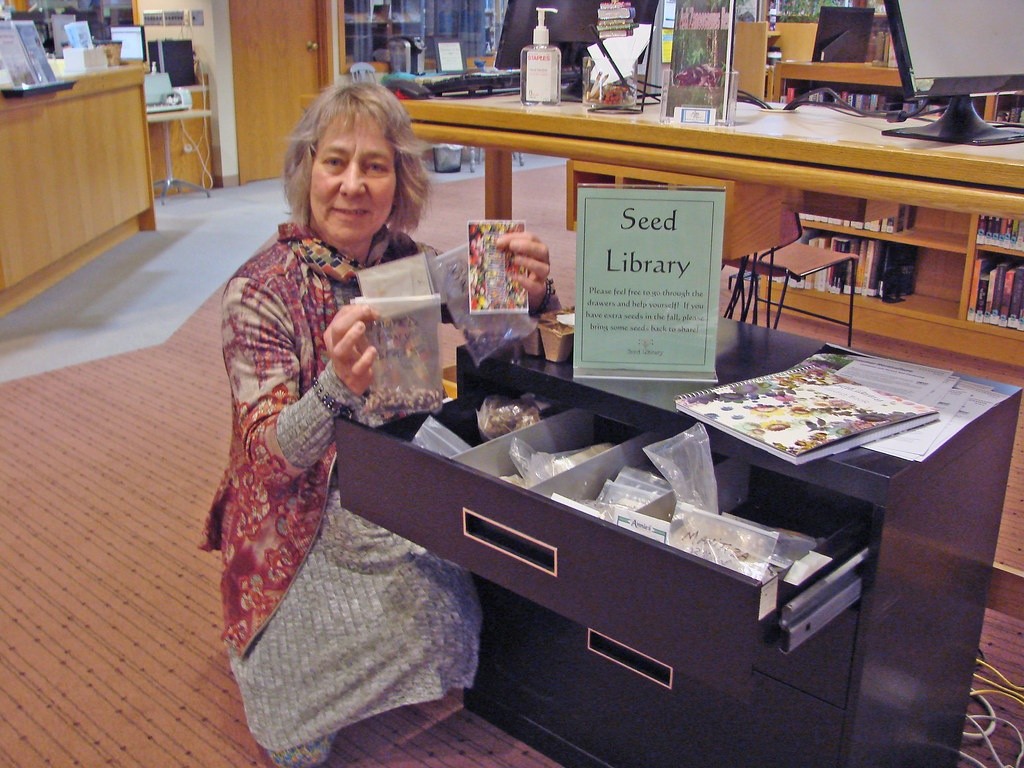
[34,37,56,82]
[198,83,561,768]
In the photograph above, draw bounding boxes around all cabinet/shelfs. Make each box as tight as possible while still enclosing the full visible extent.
[333,94,1024,768]
[775,59,902,102]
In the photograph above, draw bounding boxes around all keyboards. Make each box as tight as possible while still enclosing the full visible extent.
[423,66,584,99]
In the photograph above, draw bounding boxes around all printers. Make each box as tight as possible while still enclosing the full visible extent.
[142,71,194,114]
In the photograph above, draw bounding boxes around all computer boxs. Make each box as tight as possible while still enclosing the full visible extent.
[146,39,195,88]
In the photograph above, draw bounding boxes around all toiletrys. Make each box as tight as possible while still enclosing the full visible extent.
[521,6,562,108]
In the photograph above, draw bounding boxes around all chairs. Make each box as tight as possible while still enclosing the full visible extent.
[724,241,859,346]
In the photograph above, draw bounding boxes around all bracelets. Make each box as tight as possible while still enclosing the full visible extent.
[527,277,556,317]
[312,377,354,416]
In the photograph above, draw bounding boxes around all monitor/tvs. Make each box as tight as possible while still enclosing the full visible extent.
[106,25,146,64]
[488,0,659,104]
[881,0,1024,146]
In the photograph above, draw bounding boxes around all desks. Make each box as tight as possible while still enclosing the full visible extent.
[146,110,212,203]
[386,67,581,170]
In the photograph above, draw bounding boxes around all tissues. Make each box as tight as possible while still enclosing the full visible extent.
[61,20,109,76]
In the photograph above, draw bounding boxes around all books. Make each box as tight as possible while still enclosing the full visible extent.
[966,215,1024,331]
[780,79,945,118]
[596,1,639,39]
[665,0,736,121]
[995,94,1024,124]
[866,21,898,67]
[767,205,918,299]
[672,363,938,466]
